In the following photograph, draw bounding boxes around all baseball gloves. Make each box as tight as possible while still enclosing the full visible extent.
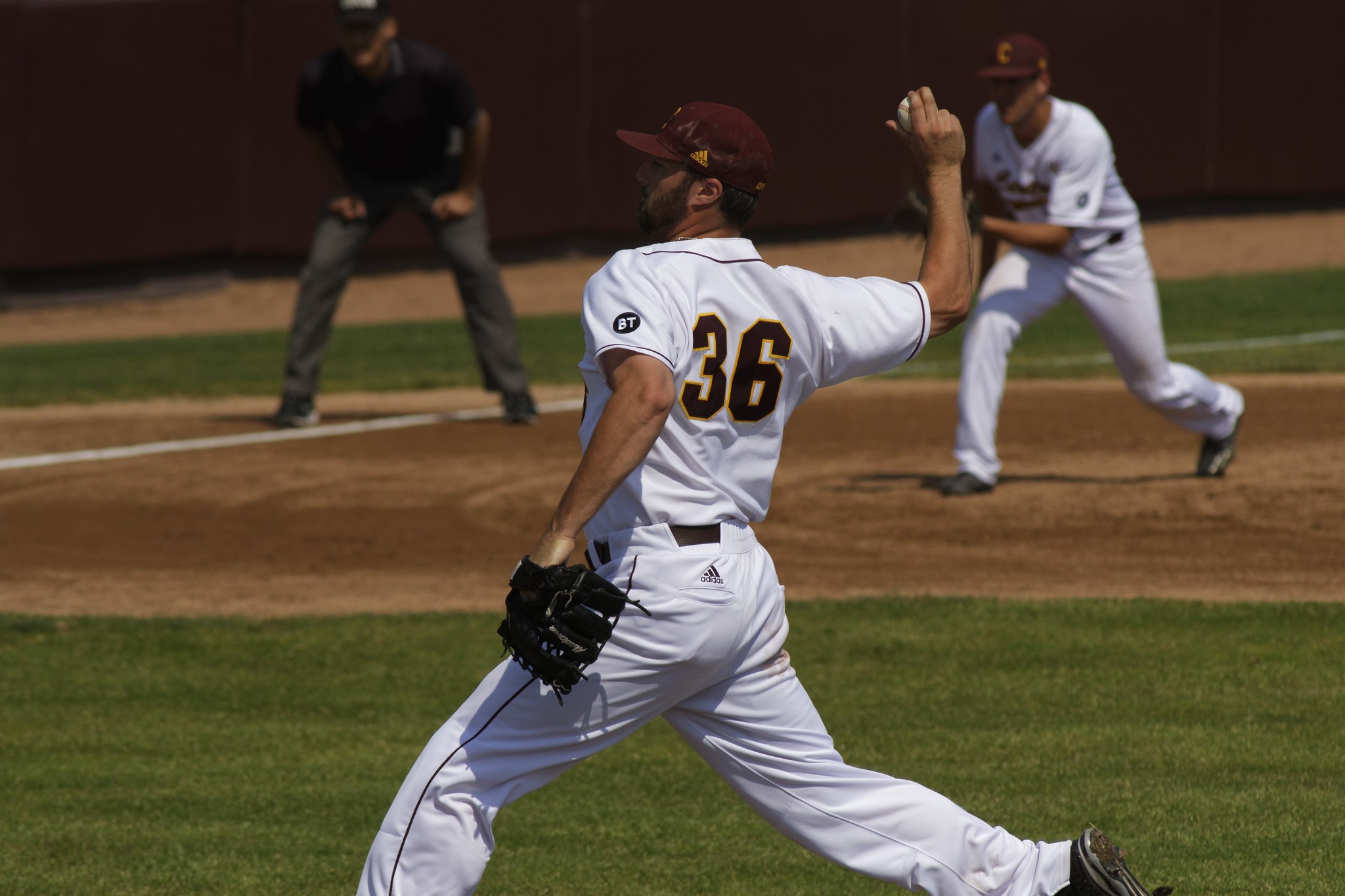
[907,181,978,239]
[495,551,654,708]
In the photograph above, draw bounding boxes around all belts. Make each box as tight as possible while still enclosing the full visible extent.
[1107,231,1122,244]
[584,525,723,570]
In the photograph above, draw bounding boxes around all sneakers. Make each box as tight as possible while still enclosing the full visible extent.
[939,472,992,494]
[1198,426,1234,476]
[501,388,541,424]
[277,393,317,425]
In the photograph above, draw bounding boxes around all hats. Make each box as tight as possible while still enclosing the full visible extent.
[331,0,384,20]
[976,29,1047,76]
[614,101,771,197]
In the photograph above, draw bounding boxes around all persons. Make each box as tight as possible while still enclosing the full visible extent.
[357,85,1151,896]
[941,37,1244,498]
[273,0,539,428]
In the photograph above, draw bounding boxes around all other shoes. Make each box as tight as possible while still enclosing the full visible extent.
[1066,822,1151,896]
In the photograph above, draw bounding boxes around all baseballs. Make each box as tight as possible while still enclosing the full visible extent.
[896,96,939,134]
